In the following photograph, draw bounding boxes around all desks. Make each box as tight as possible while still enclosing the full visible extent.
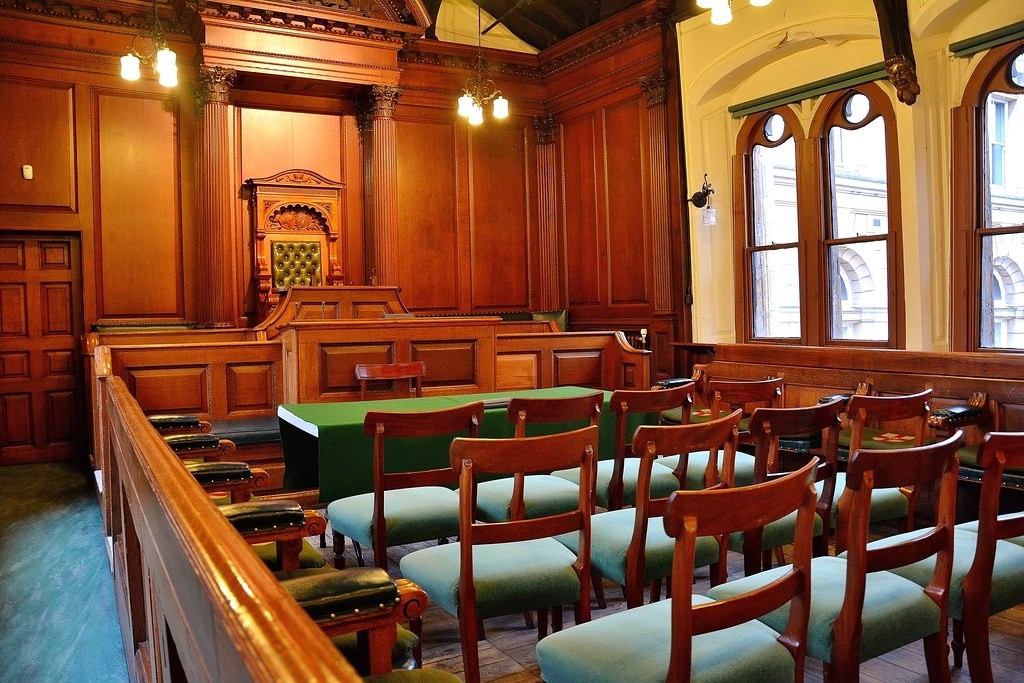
[277,385,663,571]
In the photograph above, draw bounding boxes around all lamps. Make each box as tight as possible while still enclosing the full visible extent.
[119,0,180,87]
[688,174,717,226]
[457,0,509,125]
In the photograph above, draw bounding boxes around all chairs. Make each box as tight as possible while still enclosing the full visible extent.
[138,359,1023,683]
